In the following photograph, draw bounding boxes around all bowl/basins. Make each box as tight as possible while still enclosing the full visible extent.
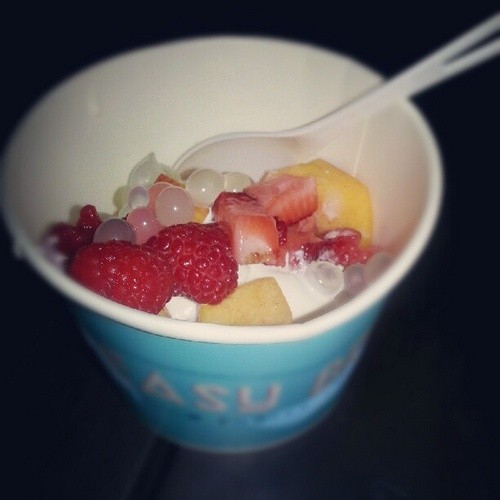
[4,35,443,455]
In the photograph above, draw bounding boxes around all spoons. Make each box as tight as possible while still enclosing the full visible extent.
[171,10,500,182]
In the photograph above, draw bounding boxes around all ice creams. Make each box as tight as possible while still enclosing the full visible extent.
[47,149,391,325]
